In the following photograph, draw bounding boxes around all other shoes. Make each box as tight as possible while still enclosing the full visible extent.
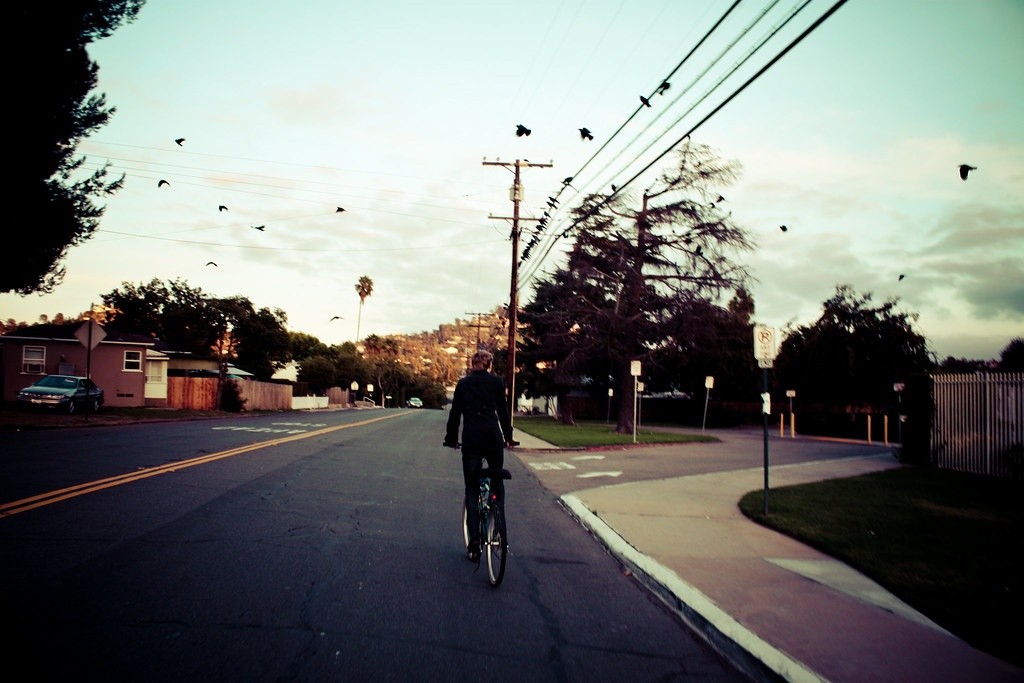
[466,540,480,554]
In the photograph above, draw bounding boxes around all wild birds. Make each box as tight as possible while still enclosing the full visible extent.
[336,206,346,213]
[578,127,594,142]
[255,224,266,232]
[958,164,978,181]
[610,184,618,192]
[329,316,344,322]
[779,225,788,232]
[219,205,228,212]
[710,195,725,208]
[157,179,171,188]
[515,123,531,137]
[561,177,573,185]
[205,261,219,268]
[175,137,185,147]
[897,274,907,281]
[639,79,671,108]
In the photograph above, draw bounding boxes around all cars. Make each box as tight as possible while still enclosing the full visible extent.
[17,375,104,414]
[407,397,423,409]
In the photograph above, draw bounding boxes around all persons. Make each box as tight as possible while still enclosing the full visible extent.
[444,350,513,560]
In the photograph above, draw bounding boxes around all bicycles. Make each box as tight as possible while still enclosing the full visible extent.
[442,433,519,590]
